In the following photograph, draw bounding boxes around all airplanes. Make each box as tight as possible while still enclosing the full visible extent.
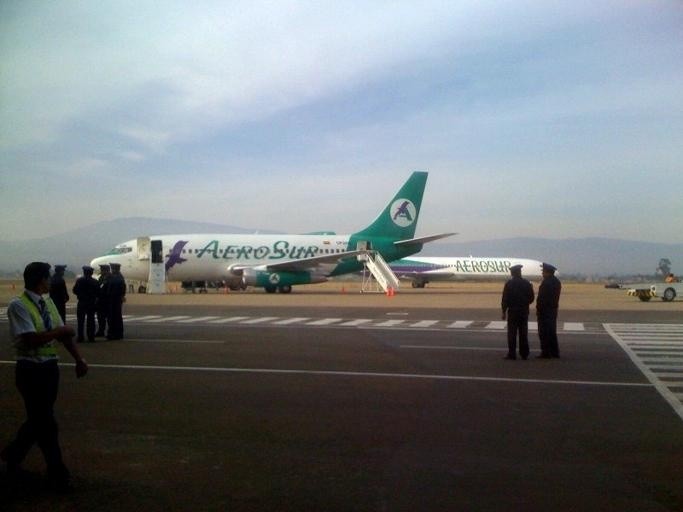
[88,169,458,293]
[354,255,559,287]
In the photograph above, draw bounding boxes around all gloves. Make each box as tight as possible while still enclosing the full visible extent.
[75,358,90,379]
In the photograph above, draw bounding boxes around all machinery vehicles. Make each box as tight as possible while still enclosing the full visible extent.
[617,280,683,301]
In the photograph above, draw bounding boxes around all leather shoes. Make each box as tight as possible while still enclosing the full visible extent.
[537,356,552,359]
[504,356,516,360]
[72,332,124,342]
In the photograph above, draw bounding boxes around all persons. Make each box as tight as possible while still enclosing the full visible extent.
[94,264,109,339]
[535,262,561,360]
[5,261,88,482]
[104,262,126,340]
[71,266,99,343]
[48,265,69,326]
[501,265,534,360]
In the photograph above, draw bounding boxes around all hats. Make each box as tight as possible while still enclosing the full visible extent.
[55,262,122,274]
[508,264,523,272]
[540,262,557,270]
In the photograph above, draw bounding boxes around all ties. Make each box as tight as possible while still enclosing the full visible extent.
[39,299,52,328]
